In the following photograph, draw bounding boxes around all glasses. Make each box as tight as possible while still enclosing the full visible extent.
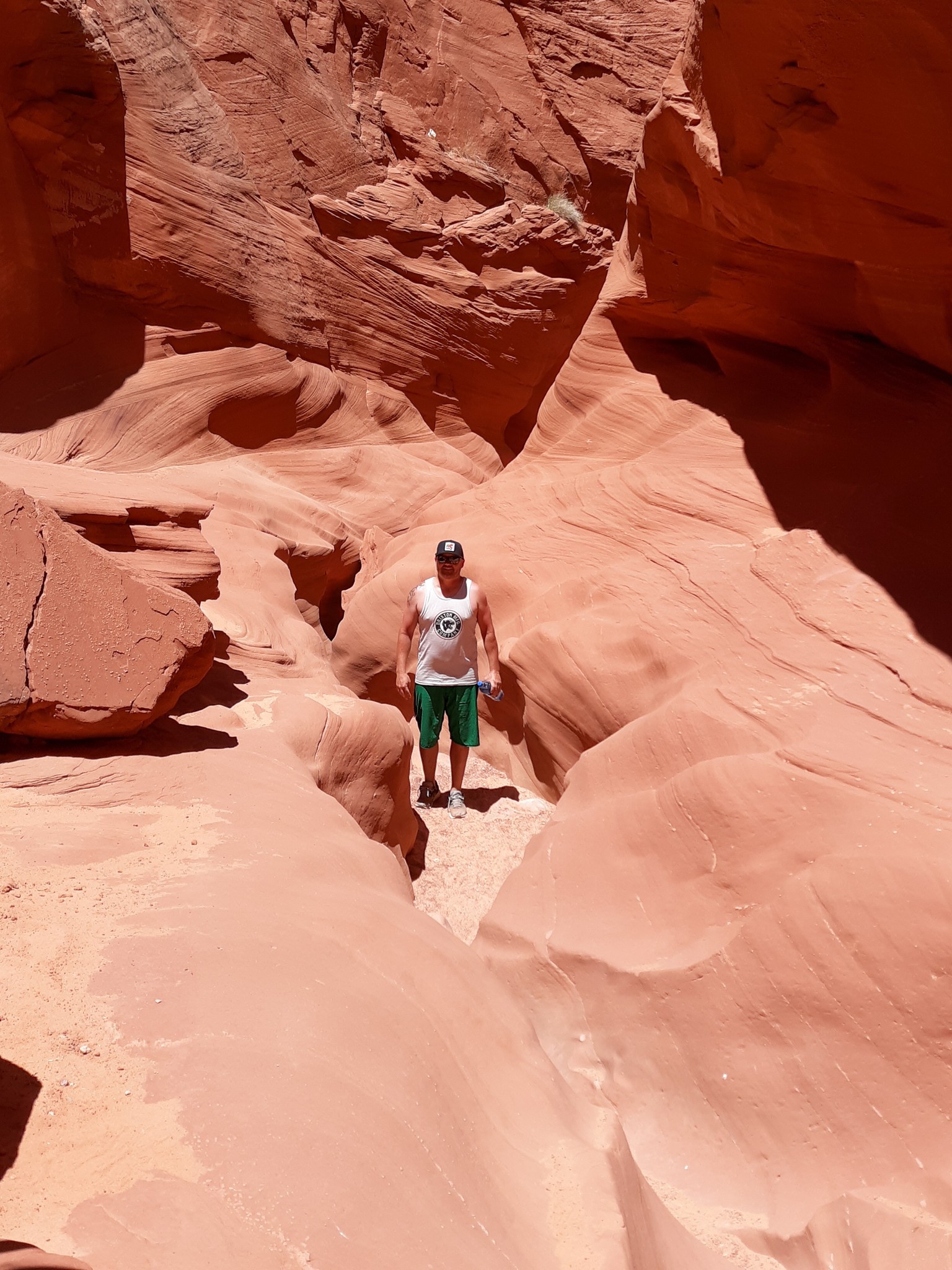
[436,554,462,564]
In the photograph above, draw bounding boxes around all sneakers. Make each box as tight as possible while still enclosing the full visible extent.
[415,781,441,809]
[447,791,467,819]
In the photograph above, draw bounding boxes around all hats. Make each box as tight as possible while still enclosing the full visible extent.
[435,540,464,559]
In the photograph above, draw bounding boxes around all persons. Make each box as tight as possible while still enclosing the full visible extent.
[396,540,502,819]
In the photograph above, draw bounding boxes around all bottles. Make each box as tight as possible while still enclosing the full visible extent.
[478,681,504,702]
